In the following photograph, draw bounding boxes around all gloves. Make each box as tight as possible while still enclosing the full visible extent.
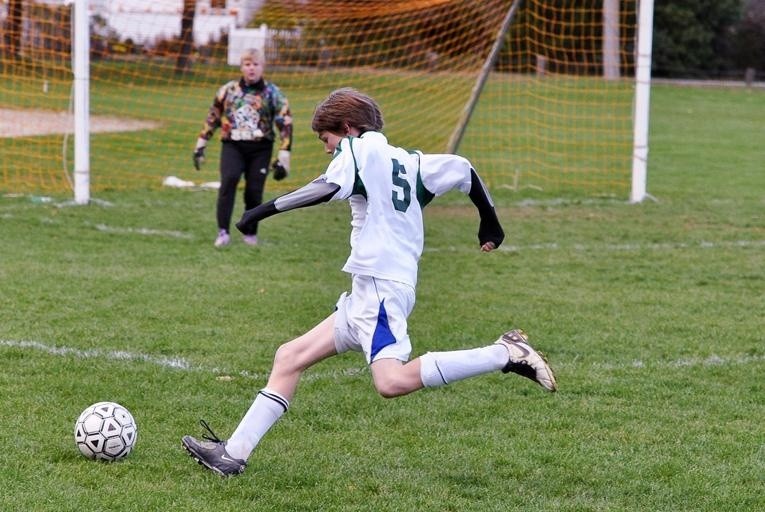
[193,147,205,170]
[271,160,287,180]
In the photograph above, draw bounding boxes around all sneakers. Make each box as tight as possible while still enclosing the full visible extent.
[494,328,558,392]
[214,228,257,247]
[180,420,247,478]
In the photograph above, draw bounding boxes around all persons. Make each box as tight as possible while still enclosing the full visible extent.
[191,45,295,249]
[179,86,558,479]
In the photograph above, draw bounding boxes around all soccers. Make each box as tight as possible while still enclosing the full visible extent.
[74,401,137,461]
